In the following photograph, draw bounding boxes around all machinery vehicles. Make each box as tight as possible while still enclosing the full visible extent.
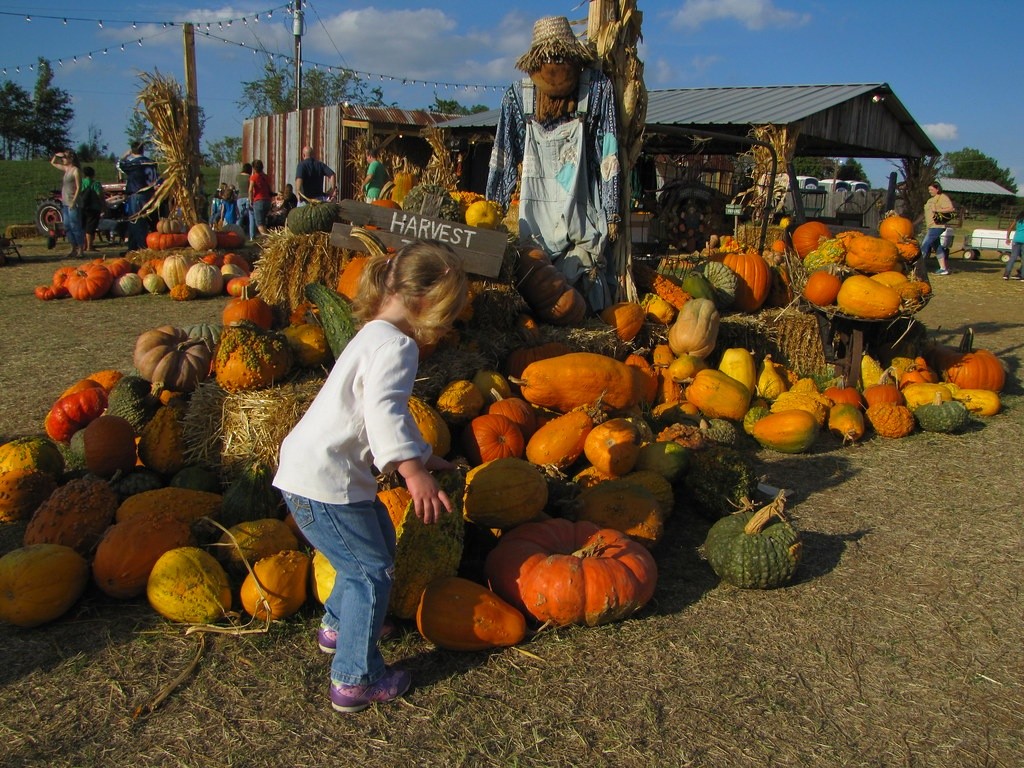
[33,182,127,239]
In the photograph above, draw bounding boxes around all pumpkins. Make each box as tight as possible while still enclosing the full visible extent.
[0,154,1007,652]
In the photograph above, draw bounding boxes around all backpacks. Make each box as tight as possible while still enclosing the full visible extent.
[80,180,100,216]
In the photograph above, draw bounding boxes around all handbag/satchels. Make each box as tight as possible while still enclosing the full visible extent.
[933,212,957,224]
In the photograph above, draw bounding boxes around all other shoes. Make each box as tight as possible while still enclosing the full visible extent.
[1019,277,1024,283]
[86,245,96,251]
[1011,275,1020,279]
[64,252,77,259]
[74,252,85,259]
[1003,274,1009,279]
[249,237,255,244]
[931,268,949,275]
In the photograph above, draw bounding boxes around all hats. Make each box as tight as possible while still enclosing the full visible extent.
[515,16,597,71]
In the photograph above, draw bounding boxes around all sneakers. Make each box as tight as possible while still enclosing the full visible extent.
[330,667,412,712]
[318,622,396,654]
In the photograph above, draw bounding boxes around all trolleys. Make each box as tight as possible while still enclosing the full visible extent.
[785,162,932,388]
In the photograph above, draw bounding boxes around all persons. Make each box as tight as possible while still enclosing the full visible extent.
[326,186,338,202]
[81,167,105,252]
[913,182,953,275]
[273,240,468,712]
[267,184,298,228]
[51,148,85,258]
[1002,210,1024,282]
[209,183,239,228]
[236,163,254,241]
[295,146,336,207]
[249,160,272,235]
[361,150,386,204]
[116,142,159,250]
